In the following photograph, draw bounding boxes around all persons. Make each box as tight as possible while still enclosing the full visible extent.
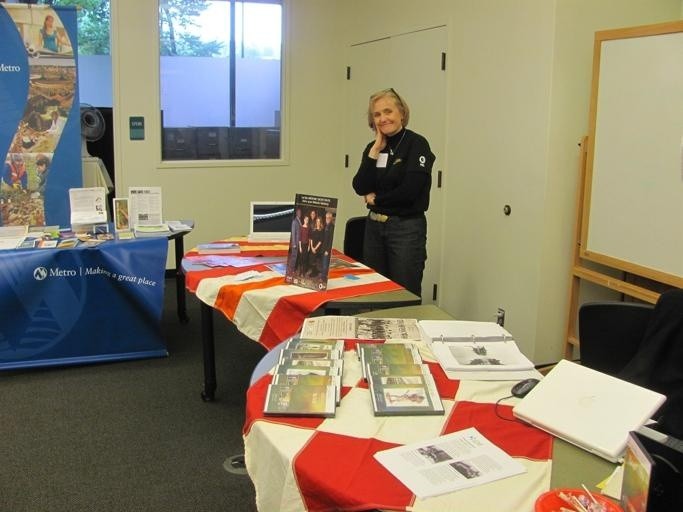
[30,155,49,198]
[287,207,333,285]
[353,87,436,296]
[1,150,26,191]
[35,14,60,55]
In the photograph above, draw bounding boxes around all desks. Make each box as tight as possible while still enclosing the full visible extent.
[178,232,422,405]
[240,303,627,511]
[0,218,192,325]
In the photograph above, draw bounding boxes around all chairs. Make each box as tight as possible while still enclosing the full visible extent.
[578,301,657,377]
[343,216,367,262]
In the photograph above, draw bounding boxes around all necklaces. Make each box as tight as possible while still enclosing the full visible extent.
[381,132,406,156]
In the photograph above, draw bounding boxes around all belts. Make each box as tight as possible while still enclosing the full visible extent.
[367,210,424,223]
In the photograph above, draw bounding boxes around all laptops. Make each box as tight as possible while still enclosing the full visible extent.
[248,201,294,243]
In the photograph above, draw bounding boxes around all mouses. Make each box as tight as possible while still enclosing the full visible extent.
[511,379,540,398]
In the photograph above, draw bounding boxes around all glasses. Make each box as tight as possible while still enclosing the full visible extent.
[368,87,403,108]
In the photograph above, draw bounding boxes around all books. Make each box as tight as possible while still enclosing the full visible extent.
[0,223,80,250]
[198,243,241,254]
[263,320,536,418]
[69,182,195,248]
